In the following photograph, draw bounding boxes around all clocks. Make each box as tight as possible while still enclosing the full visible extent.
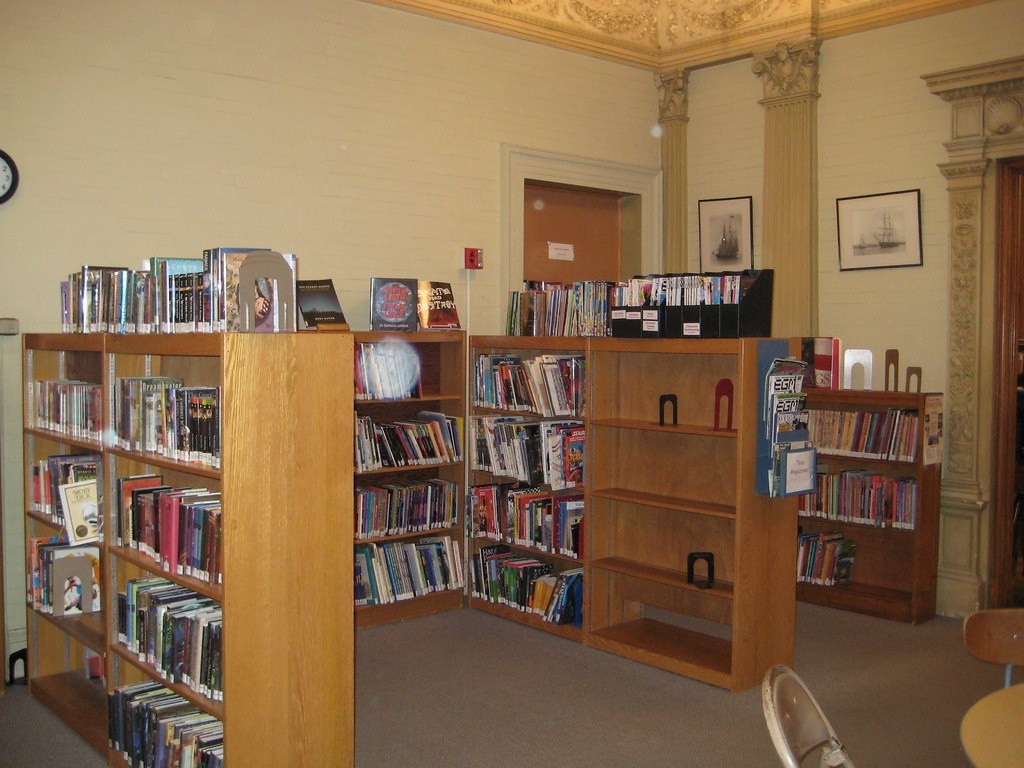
[0,149,19,204]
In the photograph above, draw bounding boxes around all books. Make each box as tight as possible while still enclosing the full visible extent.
[27,247,299,768]
[354,342,466,608]
[465,352,586,629]
[763,336,919,590]
[297,277,462,332]
[505,274,760,338]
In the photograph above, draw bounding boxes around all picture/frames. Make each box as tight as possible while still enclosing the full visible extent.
[835,188,923,271]
[698,196,754,274]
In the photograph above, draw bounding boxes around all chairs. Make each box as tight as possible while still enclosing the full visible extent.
[963,607,1024,690]
[762,662,857,768]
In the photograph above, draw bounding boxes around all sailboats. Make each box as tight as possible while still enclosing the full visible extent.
[714,216,742,259]
[872,214,899,247]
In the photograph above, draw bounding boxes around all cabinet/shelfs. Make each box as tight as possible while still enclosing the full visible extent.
[21,329,354,768]
[462,333,803,693]
[354,328,468,630]
[794,390,944,625]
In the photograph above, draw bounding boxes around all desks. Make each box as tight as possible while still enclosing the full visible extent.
[956,682,1024,768]
